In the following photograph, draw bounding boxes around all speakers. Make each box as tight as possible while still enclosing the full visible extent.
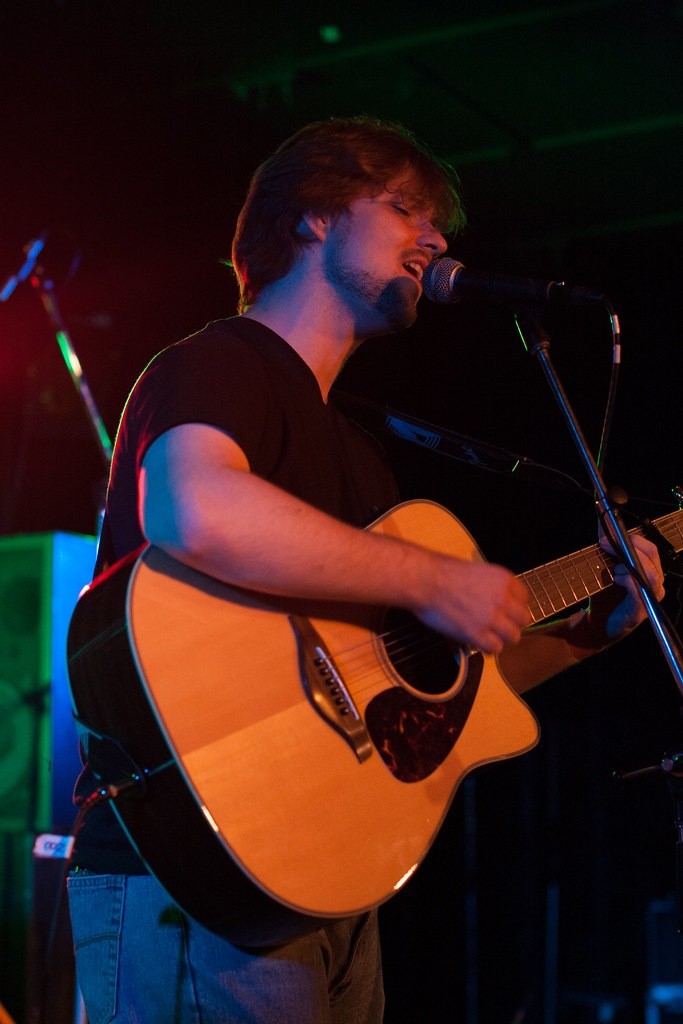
[0,531,99,832]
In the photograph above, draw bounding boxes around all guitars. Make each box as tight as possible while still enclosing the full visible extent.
[60,493,683,961]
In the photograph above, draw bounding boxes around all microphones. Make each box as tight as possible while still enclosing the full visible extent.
[422,257,605,304]
[0,238,43,301]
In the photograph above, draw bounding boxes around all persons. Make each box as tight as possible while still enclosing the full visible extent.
[67,117,665,1024]
